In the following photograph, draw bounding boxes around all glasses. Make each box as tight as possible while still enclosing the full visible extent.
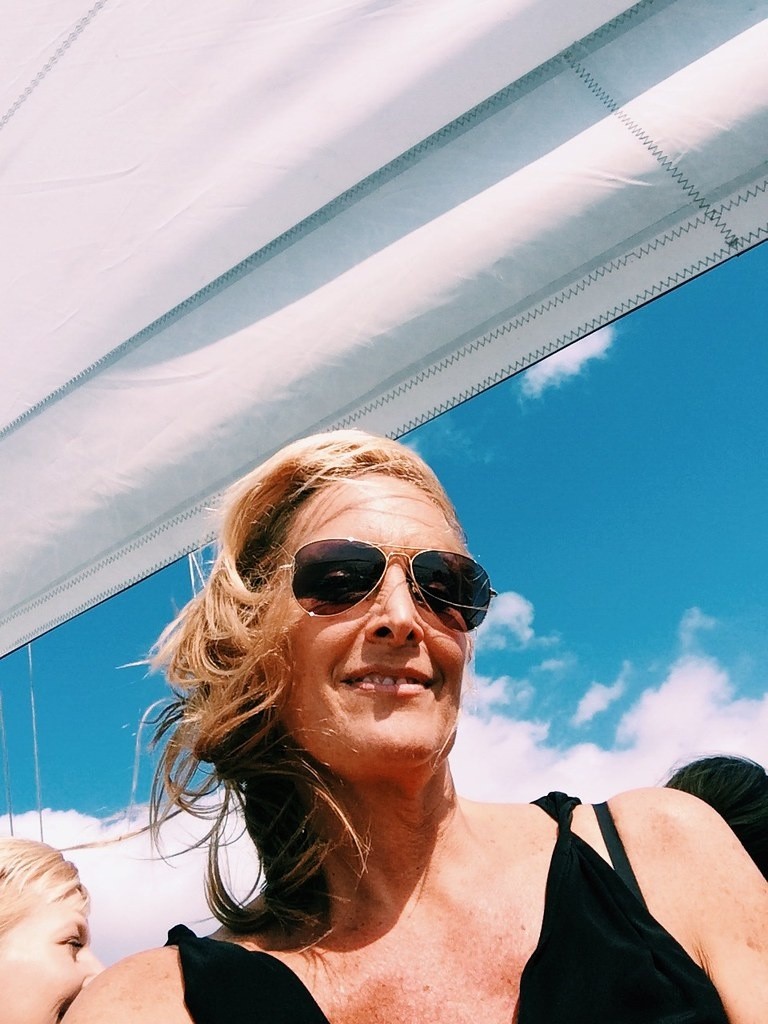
[266,539,499,634]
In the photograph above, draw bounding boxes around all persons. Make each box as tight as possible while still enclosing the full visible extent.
[664,755,768,879]
[1,839,108,1024]
[57,428,768,1024]
